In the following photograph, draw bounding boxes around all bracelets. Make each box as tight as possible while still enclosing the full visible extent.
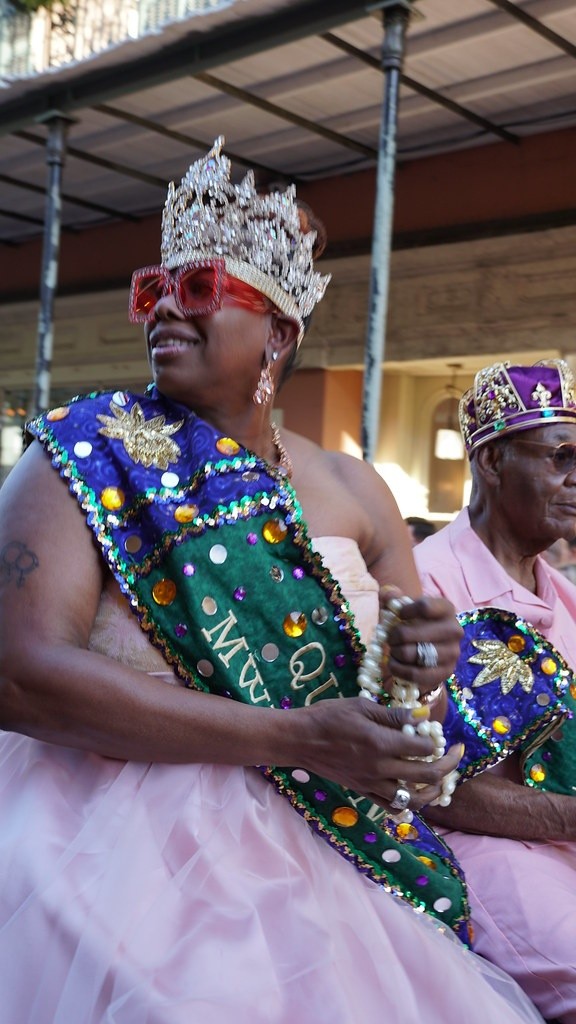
[420,682,445,707]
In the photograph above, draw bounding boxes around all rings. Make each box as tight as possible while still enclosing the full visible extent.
[390,785,410,811]
[415,643,439,667]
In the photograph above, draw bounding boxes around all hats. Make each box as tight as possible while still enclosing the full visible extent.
[458,358,576,461]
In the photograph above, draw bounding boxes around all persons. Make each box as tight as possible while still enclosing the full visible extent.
[403,357,576,1024]
[0,136,544,1023]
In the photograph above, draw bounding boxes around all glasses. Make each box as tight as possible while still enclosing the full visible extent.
[498,436,576,474]
[128,257,284,322]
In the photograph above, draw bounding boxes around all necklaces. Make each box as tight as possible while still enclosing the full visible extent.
[359,596,454,806]
[270,422,293,477]
